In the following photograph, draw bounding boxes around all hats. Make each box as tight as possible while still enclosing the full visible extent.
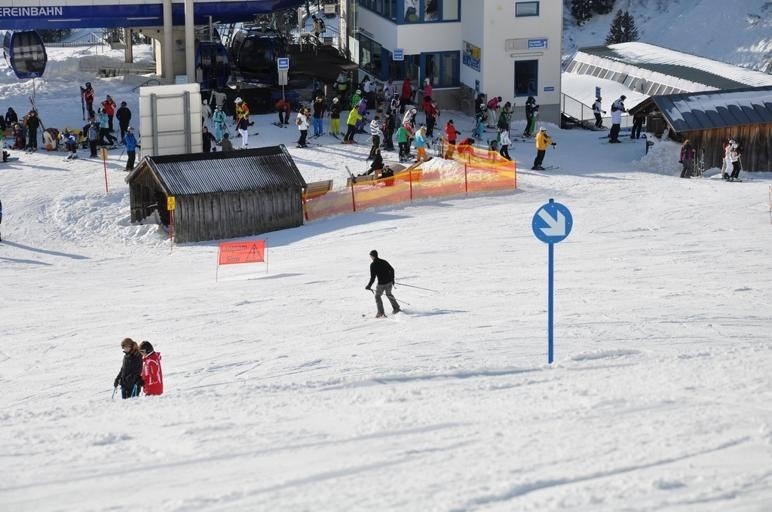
[121,338,134,345]
[138,341,153,355]
[370,250,378,258]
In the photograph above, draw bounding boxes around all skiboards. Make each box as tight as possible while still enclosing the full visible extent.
[465,121,537,151]
[604,140,637,143]
[418,121,443,131]
[271,121,287,128]
[711,176,759,182]
[599,132,631,140]
[28,96,46,129]
[293,130,356,148]
[230,132,259,137]
[80,85,86,121]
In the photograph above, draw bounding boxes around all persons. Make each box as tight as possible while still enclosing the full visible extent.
[679,140,704,178]
[631,108,646,139]
[522,96,539,136]
[720,139,741,182]
[592,97,606,127]
[276,71,475,178]
[372,54,383,74]
[203,72,255,153]
[310,13,326,38]
[608,95,627,143]
[138,341,164,396]
[472,93,513,160]
[532,126,556,170]
[112,338,143,399]
[365,250,399,318]
[0,200,2,241]
[1,80,136,171]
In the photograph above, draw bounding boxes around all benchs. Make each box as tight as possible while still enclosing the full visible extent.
[346,175,378,187]
[302,180,333,200]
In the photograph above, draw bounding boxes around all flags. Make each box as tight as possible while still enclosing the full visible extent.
[220,241,265,263]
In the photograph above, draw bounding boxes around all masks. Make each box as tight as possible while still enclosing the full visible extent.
[124,347,132,353]
